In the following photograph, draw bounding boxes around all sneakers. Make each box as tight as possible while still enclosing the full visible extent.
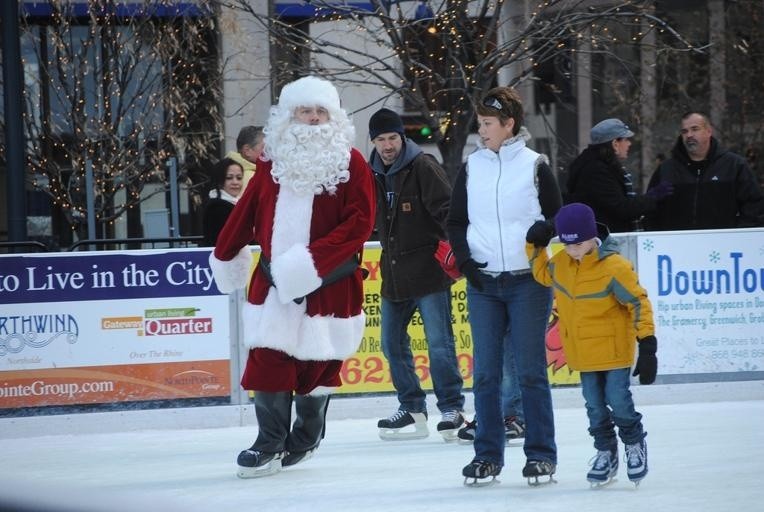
[625,439,647,480]
[504,417,526,438]
[282,443,319,466]
[237,448,283,467]
[587,449,618,482]
[523,459,556,475]
[458,415,477,441]
[462,459,501,478]
[436,410,463,430]
[378,409,427,428]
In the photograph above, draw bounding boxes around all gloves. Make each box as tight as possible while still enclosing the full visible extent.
[461,256,488,292]
[646,180,675,202]
[435,242,462,280]
[633,335,657,384]
[526,221,553,246]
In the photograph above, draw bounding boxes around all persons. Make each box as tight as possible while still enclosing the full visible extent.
[445,86,563,477]
[364,108,464,431]
[642,112,764,228]
[209,76,378,468]
[525,203,657,483]
[225,126,264,199]
[202,157,259,246]
[561,118,676,232]
[435,240,524,441]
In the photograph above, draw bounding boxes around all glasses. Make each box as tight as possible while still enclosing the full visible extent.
[484,97,504,111]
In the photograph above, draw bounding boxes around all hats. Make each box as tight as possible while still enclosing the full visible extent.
[369,109,405,141]
[279,74,341,112]
[554,203,597,244]
[590,119,635,145]
